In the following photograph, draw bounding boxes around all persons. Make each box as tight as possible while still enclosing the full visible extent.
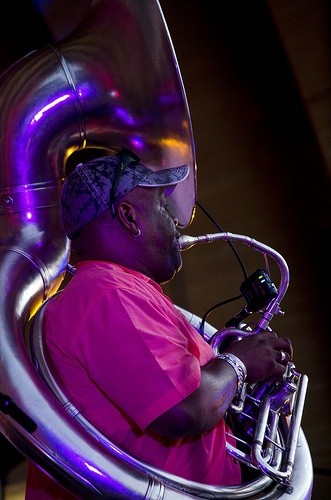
[24,154,294,500]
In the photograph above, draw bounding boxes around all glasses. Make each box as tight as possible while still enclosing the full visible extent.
[110,144,140,217]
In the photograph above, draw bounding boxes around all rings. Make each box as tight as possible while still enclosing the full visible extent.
[280,350,286,363]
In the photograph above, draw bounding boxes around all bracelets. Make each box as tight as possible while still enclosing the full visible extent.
[212,352,248,400]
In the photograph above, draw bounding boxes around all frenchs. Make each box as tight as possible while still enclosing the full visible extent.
[0,0,315,500]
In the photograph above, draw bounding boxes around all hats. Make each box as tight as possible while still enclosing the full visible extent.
[61,156,190,238]
